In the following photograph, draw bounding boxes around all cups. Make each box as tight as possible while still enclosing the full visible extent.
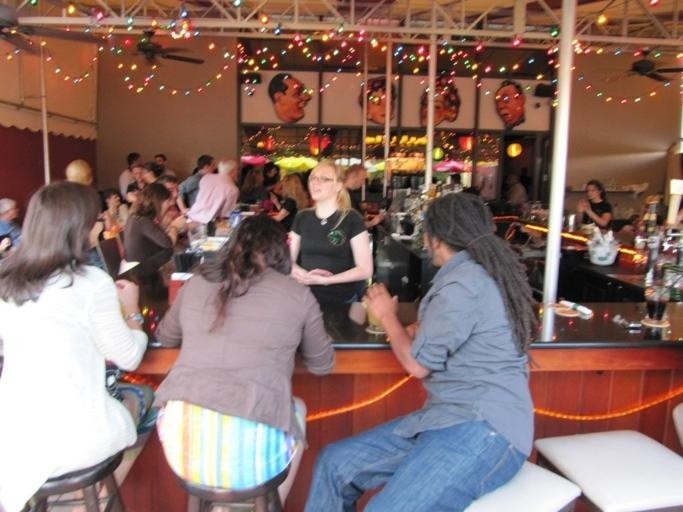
[568,214,576,226]
[569,225,574,232]
[362,293,390,334]
[644,285,670,321]
[174,251,195,271]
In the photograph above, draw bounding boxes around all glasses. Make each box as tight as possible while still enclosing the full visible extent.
[309,175,335,182]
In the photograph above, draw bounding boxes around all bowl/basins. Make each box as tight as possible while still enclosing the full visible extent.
[560,245,591,261]
[586,240,620,267]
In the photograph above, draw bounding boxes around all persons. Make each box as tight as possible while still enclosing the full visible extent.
[305,191,540,512]
[620,214,639,233]
[268,73,312,123]
[575,179,613,230]
[318,302,366,340]
[0,148,540,301]
[494,80,526,129]
[0,180,155,511]
[150,214,336,510]
[419,75,459,127]
[358,76,397,124]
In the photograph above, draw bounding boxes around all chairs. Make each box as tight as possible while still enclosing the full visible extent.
[119,231,125,243]
[96,237,120,276]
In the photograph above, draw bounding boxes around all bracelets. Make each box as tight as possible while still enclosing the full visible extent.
[124,312,145,326]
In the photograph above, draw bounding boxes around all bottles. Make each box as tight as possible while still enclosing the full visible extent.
[229,209,238,229]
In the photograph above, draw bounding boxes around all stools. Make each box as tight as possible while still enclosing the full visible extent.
[32,451,130,512]
[535,428,683,512]
[461,457,582,512]
[672,402,683,449]
[176,461,292,511]
